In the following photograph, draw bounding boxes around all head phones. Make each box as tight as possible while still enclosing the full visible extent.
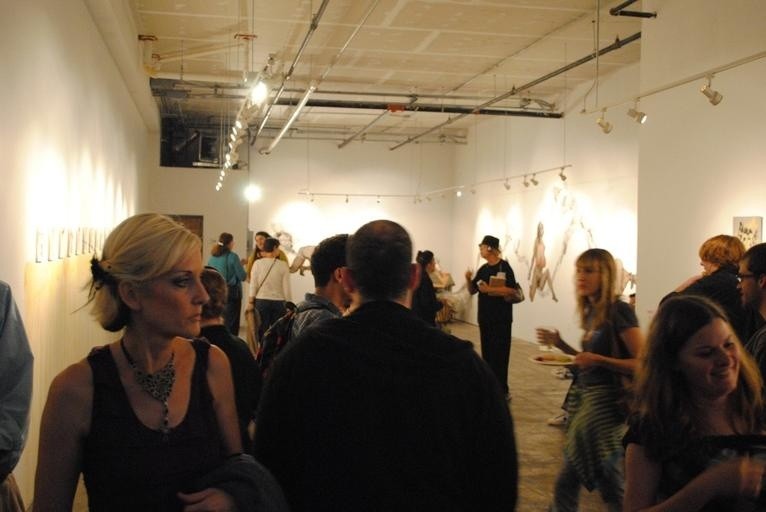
[487,246,492,251]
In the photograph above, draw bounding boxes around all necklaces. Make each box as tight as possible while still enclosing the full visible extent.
[117,336,177,426]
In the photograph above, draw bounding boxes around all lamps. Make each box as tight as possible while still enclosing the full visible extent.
[295,116,420,204]
[580,0,765,136]
[212,0,277,195]
[420,42,573,205]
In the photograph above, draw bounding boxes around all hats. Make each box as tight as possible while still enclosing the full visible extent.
[477,234,503,254]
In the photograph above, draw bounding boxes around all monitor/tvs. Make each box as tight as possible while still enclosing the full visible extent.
[197,134,226,163]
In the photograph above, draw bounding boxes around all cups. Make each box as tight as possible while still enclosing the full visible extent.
[536,324,558,353]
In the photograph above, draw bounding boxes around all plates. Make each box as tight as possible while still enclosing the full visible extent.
[529,352,577,365]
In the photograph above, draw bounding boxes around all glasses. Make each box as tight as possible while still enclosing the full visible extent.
[734,272,759,282]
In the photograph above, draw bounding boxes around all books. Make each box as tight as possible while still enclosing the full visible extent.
[487,275,507,298]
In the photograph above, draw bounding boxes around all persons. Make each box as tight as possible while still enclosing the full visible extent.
[656,232,764,350]
[291,234,354,340]
[248,232,288,278]
[545,289,638,432]
[31,210,247,511]
[618,295,765,512]
[465,235,518,401]
[250,238,290,347]
[196,269,258,458]
[253,217,522,512]
[411,250,448,330]
[210,233,246,335]
[1,279,32,512]
[535,242,648,512]
[733,242,765,396]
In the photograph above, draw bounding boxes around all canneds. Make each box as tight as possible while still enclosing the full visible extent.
[477,280,485,287]
